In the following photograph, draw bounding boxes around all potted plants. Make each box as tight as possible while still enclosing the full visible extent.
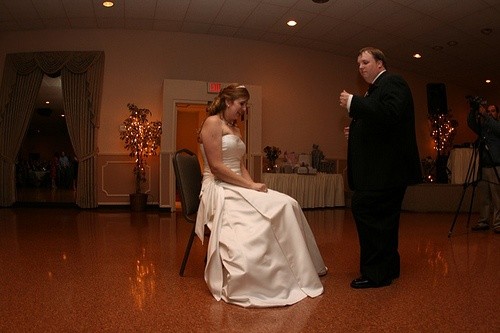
[119,103,162,214]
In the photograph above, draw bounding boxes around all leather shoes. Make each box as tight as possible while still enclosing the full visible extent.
[351,278,393,288]
[391,275,399,279]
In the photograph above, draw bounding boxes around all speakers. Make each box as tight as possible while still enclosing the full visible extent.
[426,83,447,115]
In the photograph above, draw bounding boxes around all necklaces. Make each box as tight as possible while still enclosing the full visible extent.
[219,113,235,127]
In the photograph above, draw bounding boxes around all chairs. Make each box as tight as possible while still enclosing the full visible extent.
[172,148,214,277]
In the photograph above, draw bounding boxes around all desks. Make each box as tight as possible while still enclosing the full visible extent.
[402,184,486,213]
[262,173,344,209]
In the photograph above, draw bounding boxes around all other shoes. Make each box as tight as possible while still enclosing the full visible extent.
[318,267,328,276]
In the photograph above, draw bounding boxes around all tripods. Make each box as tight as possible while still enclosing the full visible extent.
[447,131,500,238]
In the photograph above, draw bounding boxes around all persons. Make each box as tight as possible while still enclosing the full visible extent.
[194,83,328,308]
[467,103,500,233]
[339,46,424,288]
[15,151,78,191]
